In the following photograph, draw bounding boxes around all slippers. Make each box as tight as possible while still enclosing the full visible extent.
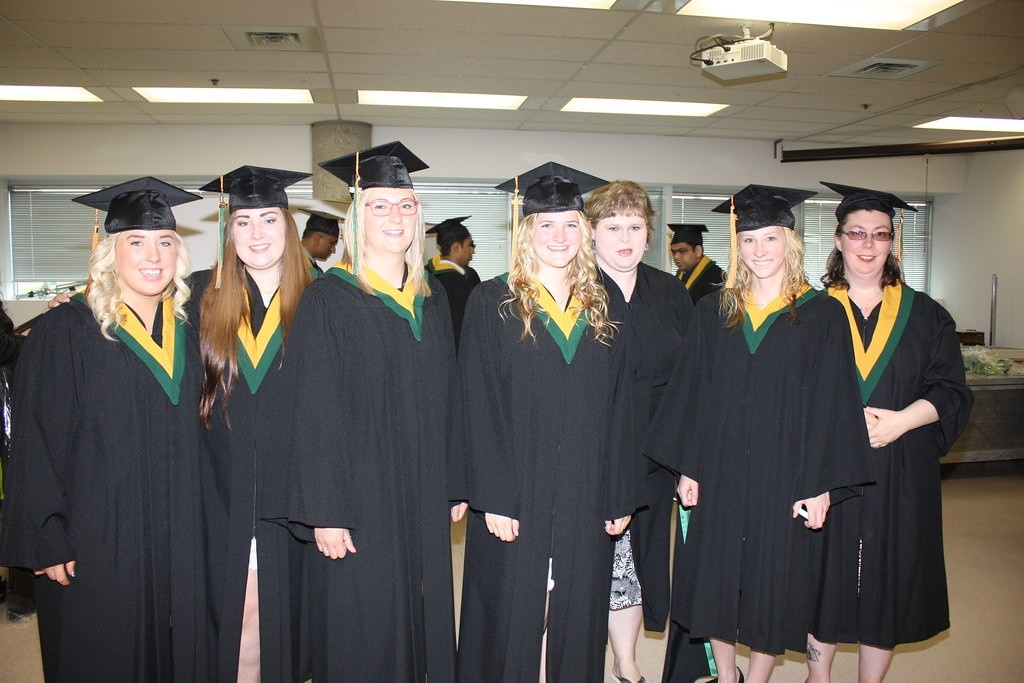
[611,673,647,683]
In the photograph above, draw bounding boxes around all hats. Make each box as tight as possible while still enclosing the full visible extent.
[493,161,610,272]
[426,216,472,243]
[300,208,344,236]
[318,141,431,278]
[711,184,819,288]
[666,223,710,256]
[72,176,204,301]
[819,181,918,283]
[200,165,313,289]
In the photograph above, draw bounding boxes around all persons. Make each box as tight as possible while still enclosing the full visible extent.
[803,181,976,683]
[0,140,728,683]
[642,184,877,683]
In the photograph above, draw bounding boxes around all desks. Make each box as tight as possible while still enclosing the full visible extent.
[939,346,1024,464]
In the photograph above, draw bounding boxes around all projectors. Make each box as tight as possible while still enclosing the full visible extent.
[701,39,788,81]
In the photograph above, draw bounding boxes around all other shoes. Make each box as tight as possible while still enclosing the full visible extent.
[718,666,745,683]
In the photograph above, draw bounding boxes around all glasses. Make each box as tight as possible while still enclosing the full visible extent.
[363,198,421,216]
[839,230,894,241]
[466,240,474,247]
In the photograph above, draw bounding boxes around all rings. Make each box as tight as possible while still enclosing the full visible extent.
[343,536,351,541]
